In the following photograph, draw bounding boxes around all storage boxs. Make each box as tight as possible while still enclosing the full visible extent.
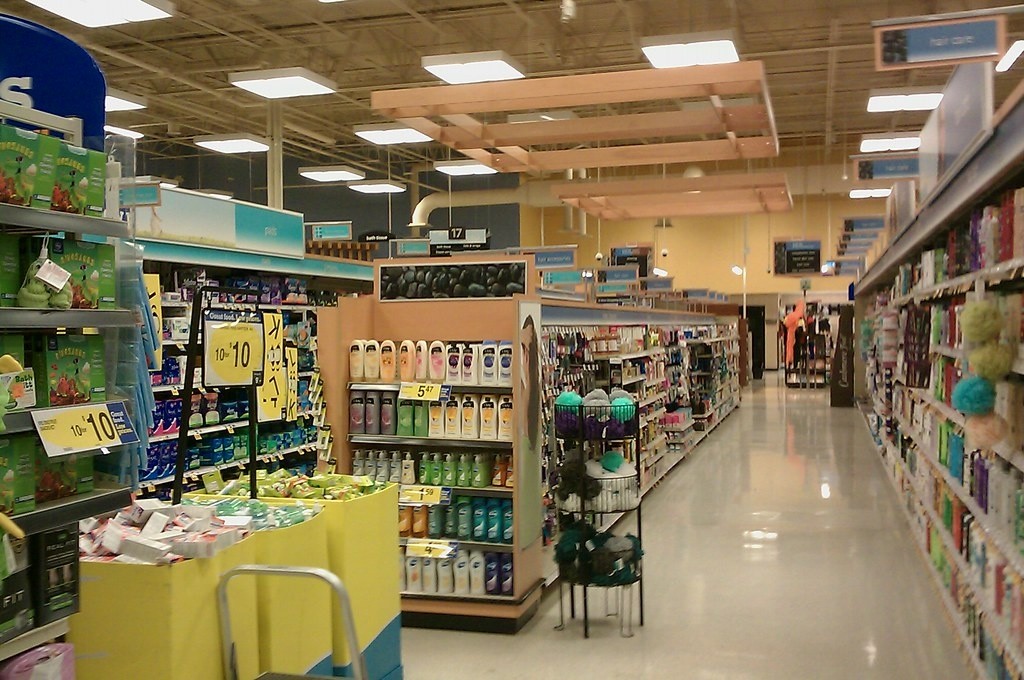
[65,471,407,680]
[0,119,117,645]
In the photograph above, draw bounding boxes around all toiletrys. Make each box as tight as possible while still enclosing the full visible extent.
[894,179,1023,680]
[349,338,513,596]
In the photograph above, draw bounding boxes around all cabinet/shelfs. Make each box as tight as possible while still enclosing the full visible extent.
[120,234,754,638]
[780,156,1024,680]
[0,98,142,662]
